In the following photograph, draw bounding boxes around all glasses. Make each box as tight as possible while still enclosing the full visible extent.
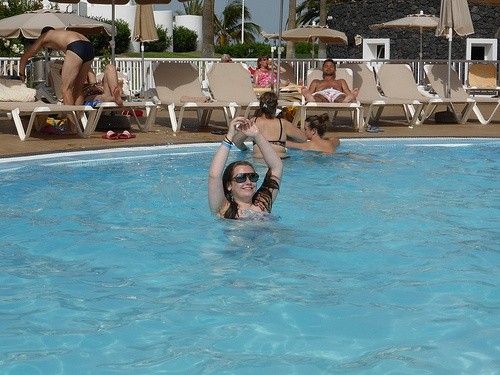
[264,59,268,61]
[227,60,232,62]
[233,173,259,183]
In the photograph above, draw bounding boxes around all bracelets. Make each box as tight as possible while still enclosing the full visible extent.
[220,141,232,149]
[224,139,231,144]
[19,72,25,76]
[223,135,233,145]
[242,147,247,151]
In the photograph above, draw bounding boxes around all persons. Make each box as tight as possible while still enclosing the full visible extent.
[19,26,95,135]
[221,53,232,62]
[59,64,124,108]
[253,55,303,88]
[232,92,308,165]
[208,117,283,220]
[300,59,359,103]
[285,113,340,153]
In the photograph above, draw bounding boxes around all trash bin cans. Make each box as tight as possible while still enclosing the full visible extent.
[34,57,50,83]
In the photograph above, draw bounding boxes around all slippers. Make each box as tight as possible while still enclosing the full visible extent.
[102,130,118,139]
[119,130,135,139]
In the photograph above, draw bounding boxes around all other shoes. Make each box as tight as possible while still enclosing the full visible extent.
[366,126,386,132]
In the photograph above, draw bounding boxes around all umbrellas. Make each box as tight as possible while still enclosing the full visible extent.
[133,0,160,99]
[0,9,117,87]
[261,24,348,68]
[434,0,475,94]
[367,10,440,85]
[40,0,193,67]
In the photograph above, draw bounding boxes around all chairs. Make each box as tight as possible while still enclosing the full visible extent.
[0,62,500,142]
